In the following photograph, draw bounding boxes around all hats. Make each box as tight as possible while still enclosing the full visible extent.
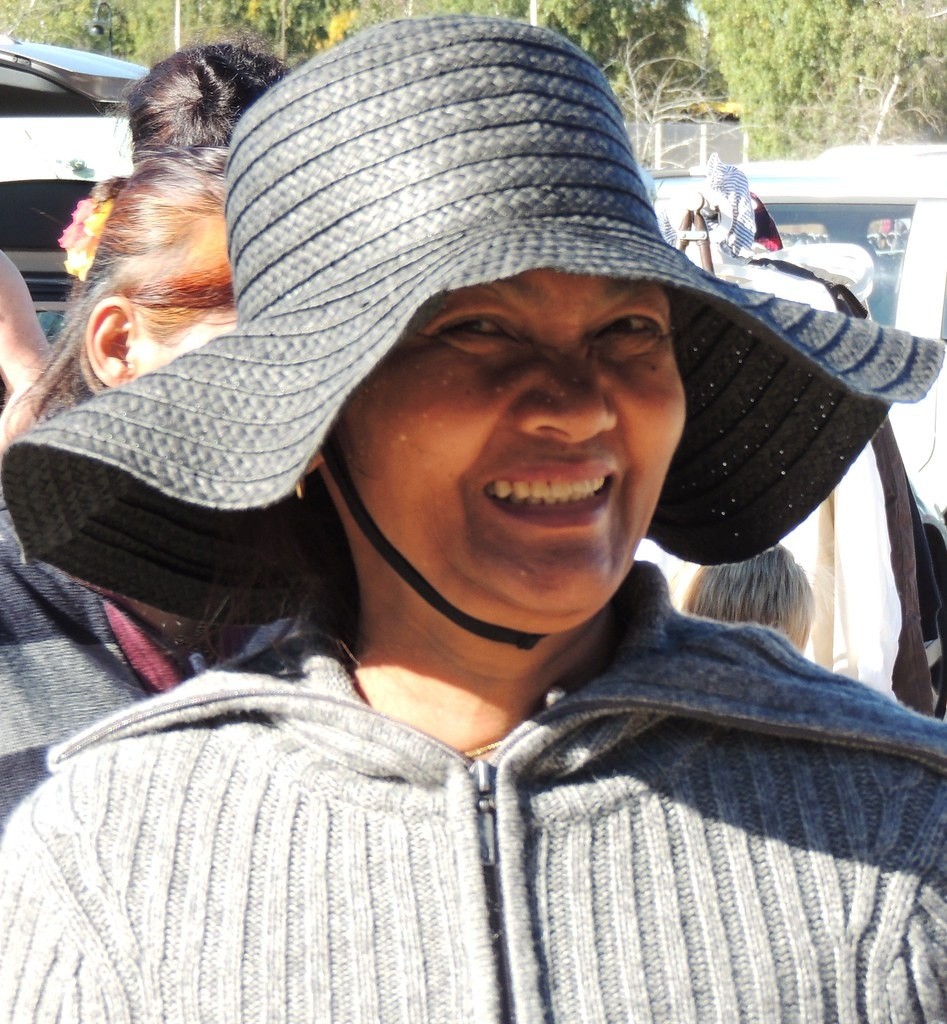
[1,16,945,619]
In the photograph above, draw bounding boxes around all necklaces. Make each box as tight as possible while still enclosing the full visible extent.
[449,739,507,768]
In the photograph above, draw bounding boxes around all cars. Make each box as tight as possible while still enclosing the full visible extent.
[0,34,150,341]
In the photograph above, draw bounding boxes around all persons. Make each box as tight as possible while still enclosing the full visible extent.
[0,41,304,838]
[656,535,816,679]
[0,16,947,1024]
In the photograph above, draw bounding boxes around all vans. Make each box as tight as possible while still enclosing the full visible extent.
[646,141,946,535]
